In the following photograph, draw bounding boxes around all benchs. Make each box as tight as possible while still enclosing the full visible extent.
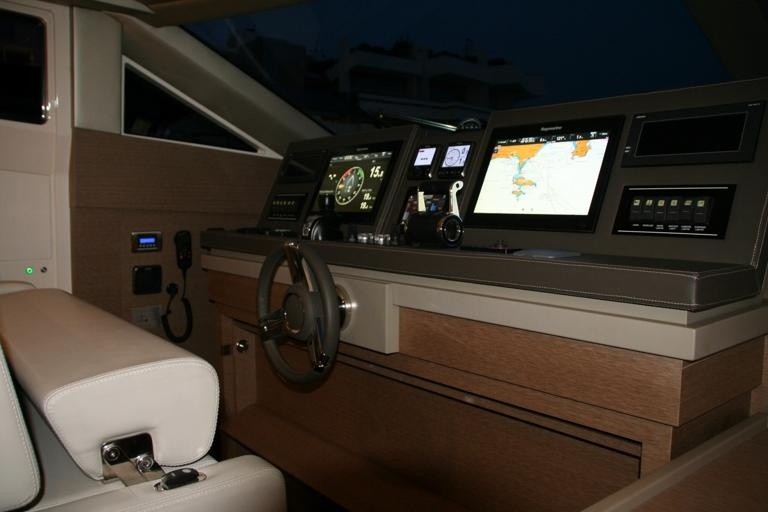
[1,281,287,512]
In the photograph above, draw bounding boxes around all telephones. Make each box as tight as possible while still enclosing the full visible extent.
[174,231,192,268]
